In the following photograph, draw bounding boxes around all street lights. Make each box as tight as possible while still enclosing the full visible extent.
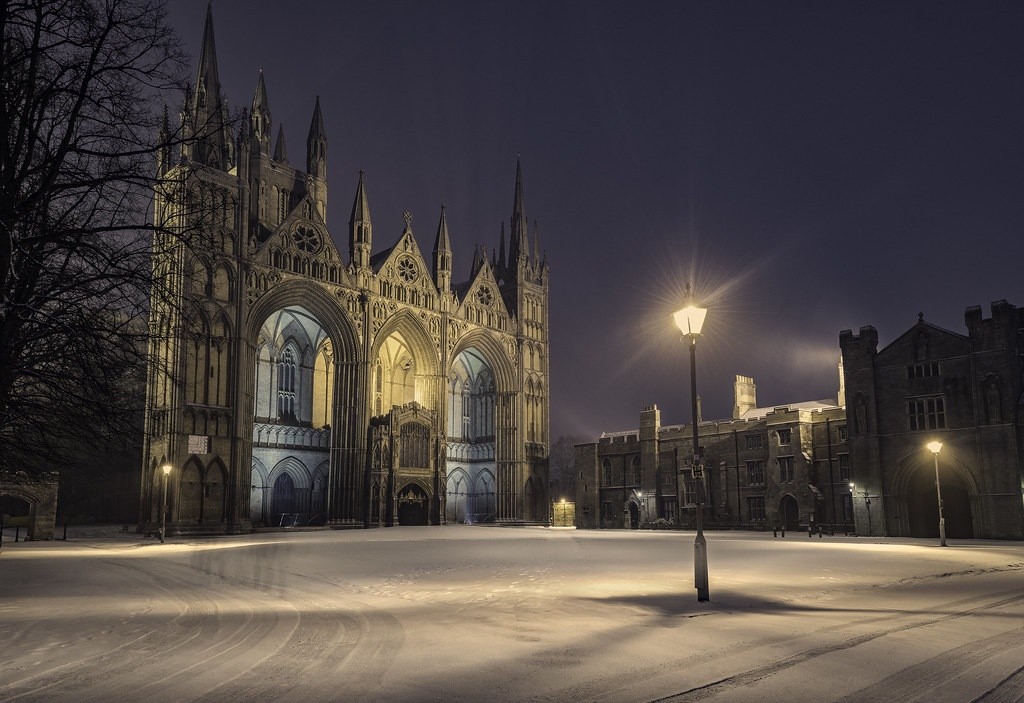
[672,283,710,604]
[926,441,947,546]
[161,465,172,543]
